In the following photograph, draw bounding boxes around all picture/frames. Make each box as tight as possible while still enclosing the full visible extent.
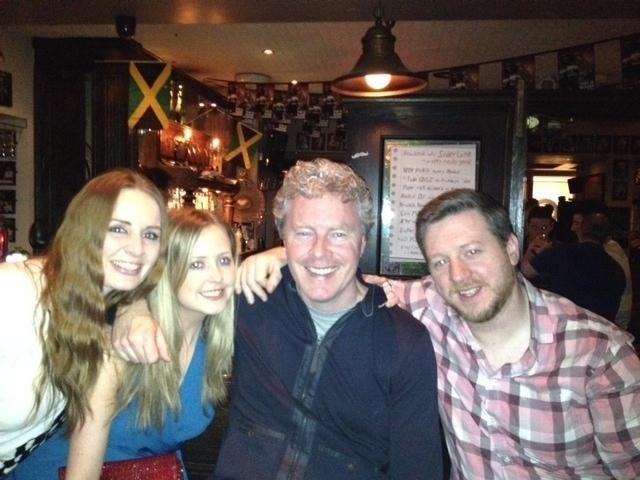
[526,134,640,201]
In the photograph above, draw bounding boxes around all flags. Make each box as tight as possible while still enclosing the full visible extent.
[224,122,262,169]
[127,63,170,129]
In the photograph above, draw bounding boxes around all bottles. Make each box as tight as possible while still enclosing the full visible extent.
[234,228,242,253]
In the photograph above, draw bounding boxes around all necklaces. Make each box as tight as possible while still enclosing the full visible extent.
[175,310,202,362]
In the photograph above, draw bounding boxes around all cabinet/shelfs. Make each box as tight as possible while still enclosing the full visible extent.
[32,34,267,260]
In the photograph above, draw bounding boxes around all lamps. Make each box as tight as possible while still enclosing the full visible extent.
[327,0,429,99]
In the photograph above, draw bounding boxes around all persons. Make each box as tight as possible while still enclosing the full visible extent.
[4,209,400,480]
[515,196,633,330]
[0,168,169,480]
[111,158,444,480]
[234,189,640,480]
